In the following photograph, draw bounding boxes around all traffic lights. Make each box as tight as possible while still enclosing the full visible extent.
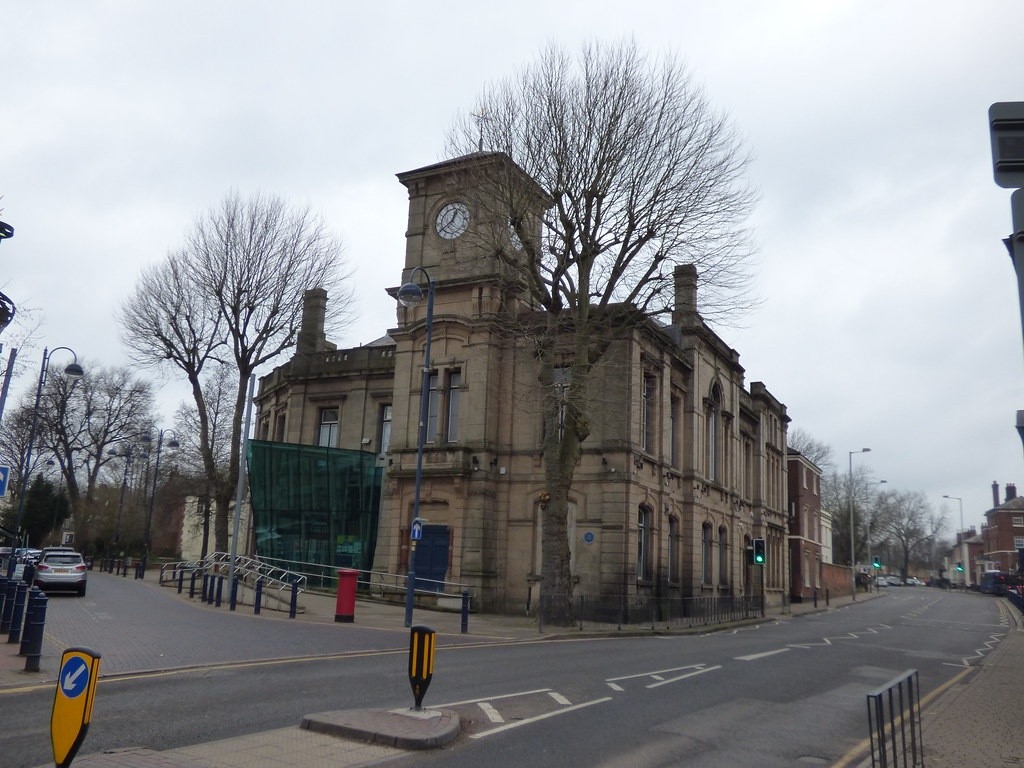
[754,539,765,564]
[957,563,962,573]
[873,556,880,570]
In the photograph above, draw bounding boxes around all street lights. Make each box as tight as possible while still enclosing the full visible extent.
[8,346,85,580]
[107,441,150,574]
[866,480,887,592]
[395,267,435,627]
[45,450,66,549]
[943,496,965,589]
[227,373,256,604]
[139,426,180,580]
[848,448,871,602]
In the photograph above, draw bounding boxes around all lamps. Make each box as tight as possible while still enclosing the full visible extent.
[361,437,370,443]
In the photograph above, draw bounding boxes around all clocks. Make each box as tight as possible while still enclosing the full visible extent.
[434,202,470,240]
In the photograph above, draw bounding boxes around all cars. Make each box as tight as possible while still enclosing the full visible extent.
[875,576,920,587]
[0,546,91,597]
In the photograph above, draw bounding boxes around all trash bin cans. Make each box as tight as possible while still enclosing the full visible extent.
[85,556,93,570]
